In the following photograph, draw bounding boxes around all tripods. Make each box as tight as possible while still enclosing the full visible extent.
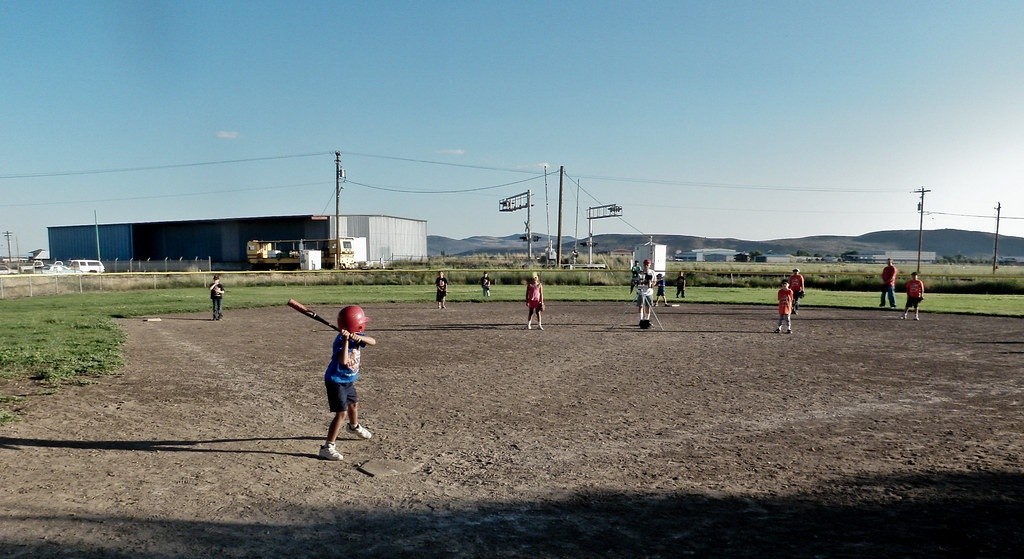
[610,284,664,330]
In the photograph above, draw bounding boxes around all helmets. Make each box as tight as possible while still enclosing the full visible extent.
[337,304,369,334]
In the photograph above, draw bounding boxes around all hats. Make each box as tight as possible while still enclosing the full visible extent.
[213,275,219,280]
[644,259,651,265]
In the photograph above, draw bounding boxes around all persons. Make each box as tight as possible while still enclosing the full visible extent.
[637,259,656,319]
[526,276,545,331]
[481,271,490,296]
[630,261,641,294]
[676,271,685,298]
[789,269,804,314]
[654,274,668,307]
[435,271,447,309]
[775,279,793,334]
[879,258,896,307]
[900,272,924,320]
[209,275,224,321]
[318,305,376,460]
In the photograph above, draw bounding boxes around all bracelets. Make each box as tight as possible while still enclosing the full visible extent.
[345,339,349,341]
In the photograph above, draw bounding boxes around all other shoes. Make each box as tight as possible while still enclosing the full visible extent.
[442,305,446,308]
[438,306,441,308]
[891,306,894,309]
[901,315,906,319]
[538,326,545,331]
[787,329,793,334]
[774,328,780,333]
[527,325,532,330]
[914,316,919,321]
[213,318,222,321]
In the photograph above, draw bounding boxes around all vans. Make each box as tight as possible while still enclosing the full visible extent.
[66,259,106,274]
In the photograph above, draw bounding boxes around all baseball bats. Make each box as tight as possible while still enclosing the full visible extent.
[286,297,367,347]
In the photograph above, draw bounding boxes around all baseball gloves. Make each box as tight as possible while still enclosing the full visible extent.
[539,303,545,312]
[799,291,805,299]
[916,296,924,303]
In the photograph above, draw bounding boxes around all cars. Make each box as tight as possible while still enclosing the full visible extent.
[999,258,1017,266]
[34,261,64,274]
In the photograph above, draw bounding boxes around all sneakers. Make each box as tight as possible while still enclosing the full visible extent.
[345,421,372,440]
[319,443,343,461]
